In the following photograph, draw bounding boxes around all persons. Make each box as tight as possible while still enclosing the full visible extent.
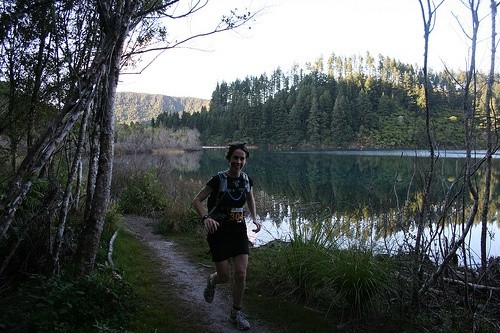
[193,141,262,331]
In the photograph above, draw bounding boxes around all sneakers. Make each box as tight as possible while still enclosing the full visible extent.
[229,310,250,330]
[204,274,216,303]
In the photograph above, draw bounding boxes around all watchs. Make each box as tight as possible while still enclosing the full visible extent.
[201,214,209,222]
